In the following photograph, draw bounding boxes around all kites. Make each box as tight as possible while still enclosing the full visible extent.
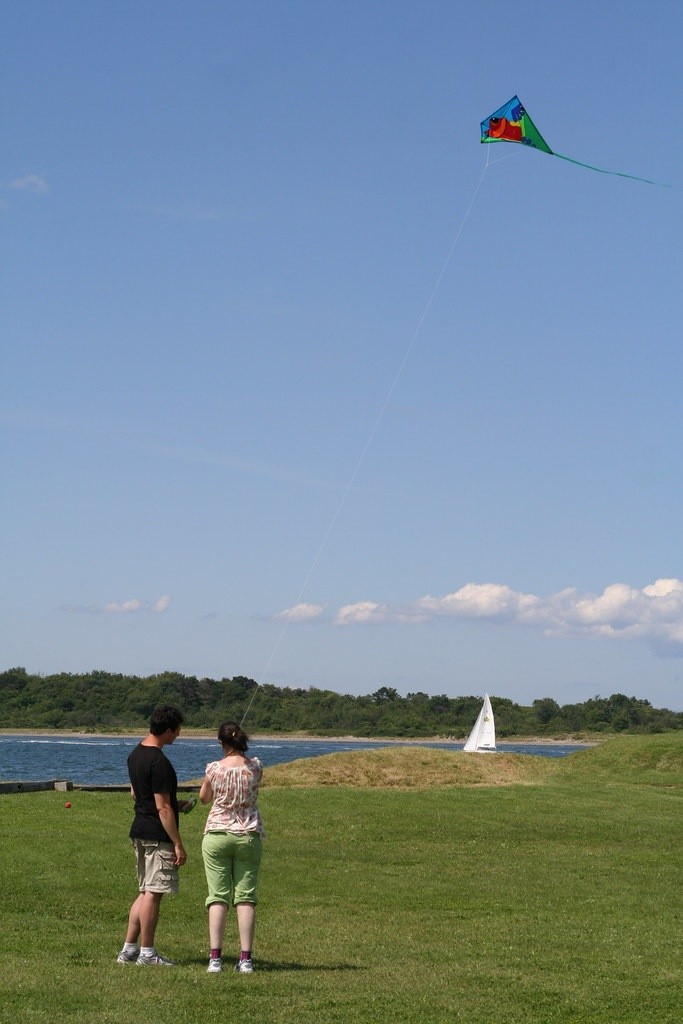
[480,95,675,190]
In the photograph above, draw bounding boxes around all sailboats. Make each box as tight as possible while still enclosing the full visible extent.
[462,691,502,753]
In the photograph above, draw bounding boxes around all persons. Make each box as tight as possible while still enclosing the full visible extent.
[198,721,263,972]
[115,705,192,967]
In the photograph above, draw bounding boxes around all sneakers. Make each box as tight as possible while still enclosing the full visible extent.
[234,958,253,973]
[135,950,173,968]
[117,950,141,962]
[208,956,223,974]
